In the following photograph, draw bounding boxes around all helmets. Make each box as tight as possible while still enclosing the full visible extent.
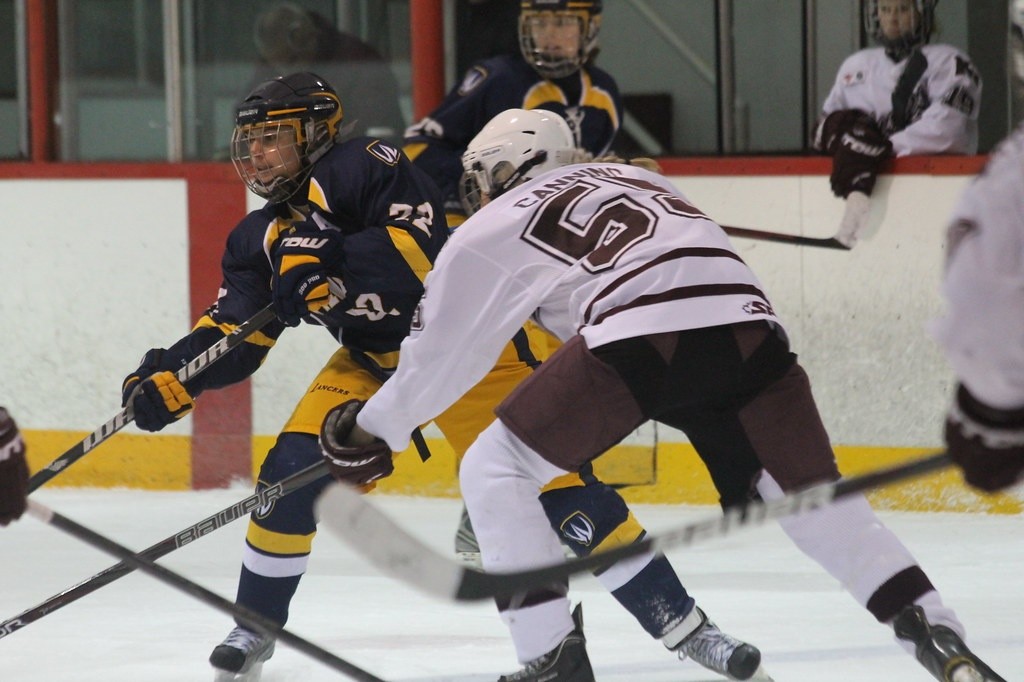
[1006,0,1024,81]
[863,0,942,62]
[458,108,577,218]
[517,0,600,78]
[229,71,344,204]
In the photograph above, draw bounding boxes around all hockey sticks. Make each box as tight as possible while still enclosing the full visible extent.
[312,450,956,604]
[0,459,333,640]
[24,304,276,497]
[23,500,382,682]
[715,191,870,250]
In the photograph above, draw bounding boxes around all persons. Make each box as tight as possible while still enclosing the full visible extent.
[237,3,406,144]
[317,108,1007,682]
[815,0,986,199]
[943,0,1024,495]
[395,0,623,572]
[122,72,777,682]
[0,406,30,526]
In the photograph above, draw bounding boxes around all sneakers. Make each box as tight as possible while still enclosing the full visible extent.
[661,602,773,682]
[498,602,596,682]
[209,624,277,682]
[892,605,1006,682]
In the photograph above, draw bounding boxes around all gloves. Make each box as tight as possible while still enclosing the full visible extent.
[320,393,395,493]
[831,114,892,200]
[0,406,31,527]
[821,110,868,149]
[264,228,345,325]
[121,347,200,432]
[945,383,1024,489]
